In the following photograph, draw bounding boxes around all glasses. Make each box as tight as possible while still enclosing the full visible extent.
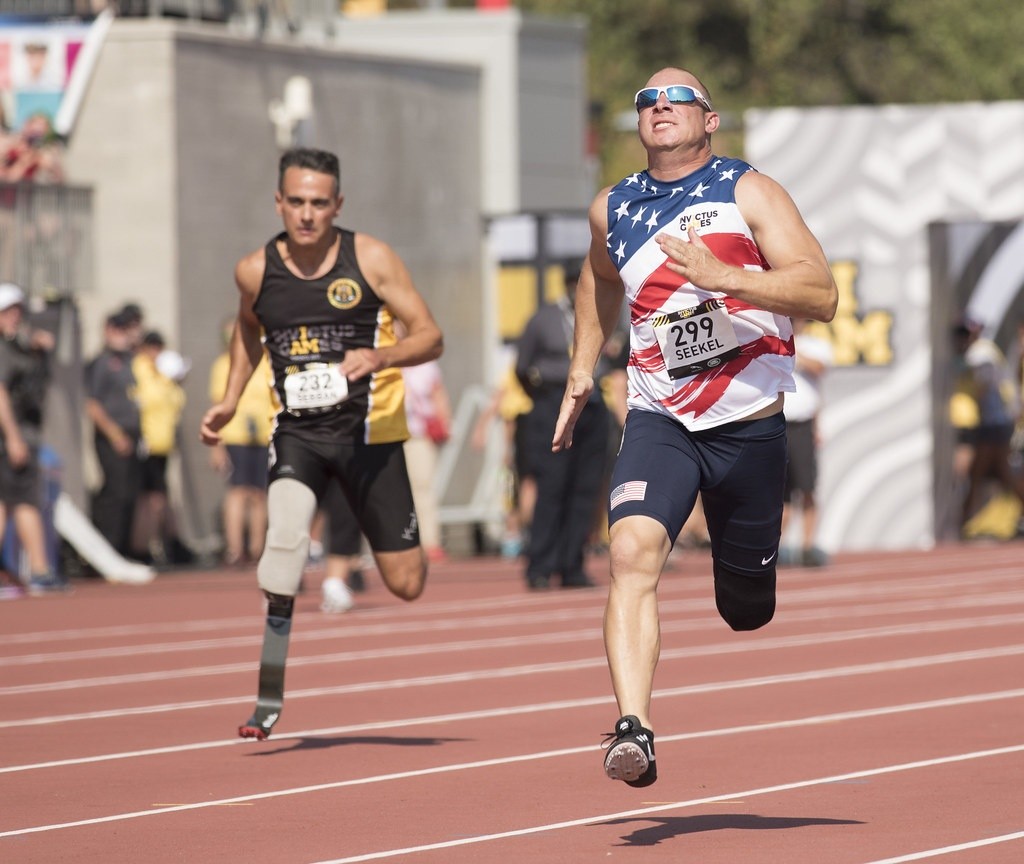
[634,84,712,113]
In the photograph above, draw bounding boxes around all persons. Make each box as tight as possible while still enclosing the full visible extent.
[199,148,444,741]
[552,66,839,789]
[948,317,1020,541]
[515,255,830,593]
[0,281,450,614]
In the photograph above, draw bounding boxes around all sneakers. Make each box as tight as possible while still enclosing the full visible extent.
[600,715,658,788]
